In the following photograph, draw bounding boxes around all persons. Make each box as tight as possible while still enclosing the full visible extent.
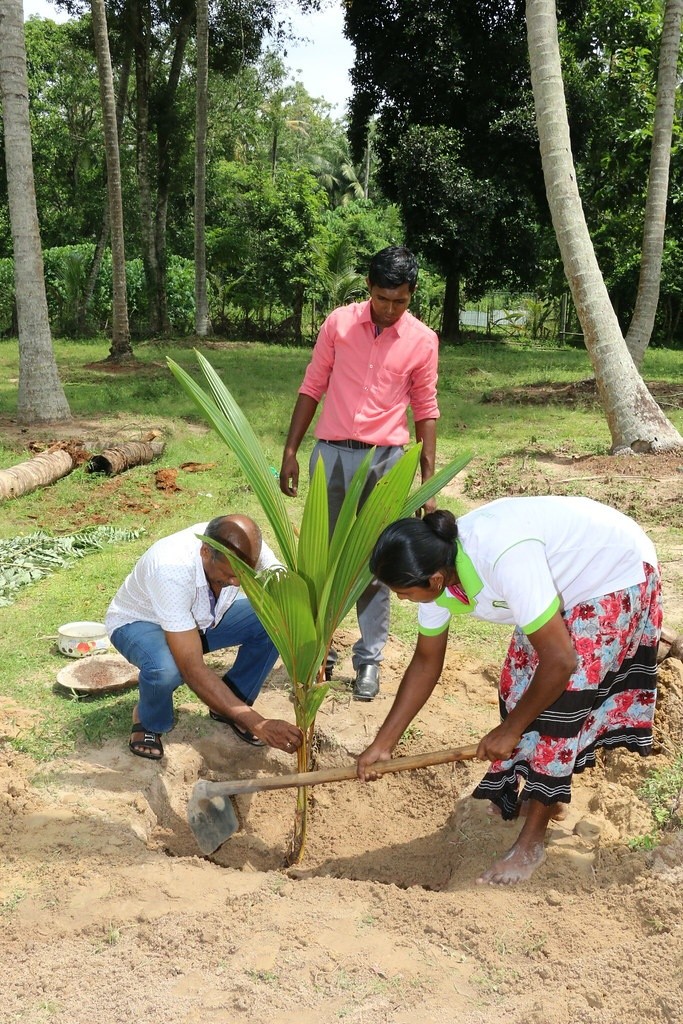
[358,495,663,886]
[279,246,440,700]
[107,514,305,760]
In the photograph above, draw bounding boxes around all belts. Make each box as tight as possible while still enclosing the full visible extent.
[320,439,380,449]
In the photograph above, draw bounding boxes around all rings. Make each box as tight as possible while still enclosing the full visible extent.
[287,743,291,747]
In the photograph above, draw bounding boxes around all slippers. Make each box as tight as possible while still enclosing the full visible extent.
[209,711,267,747]
[130,723,165,759]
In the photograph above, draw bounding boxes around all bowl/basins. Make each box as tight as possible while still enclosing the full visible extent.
[57,622,111,658]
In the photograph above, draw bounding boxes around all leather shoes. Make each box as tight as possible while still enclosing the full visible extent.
[291,650,332,700]
[353,664,380,699]
